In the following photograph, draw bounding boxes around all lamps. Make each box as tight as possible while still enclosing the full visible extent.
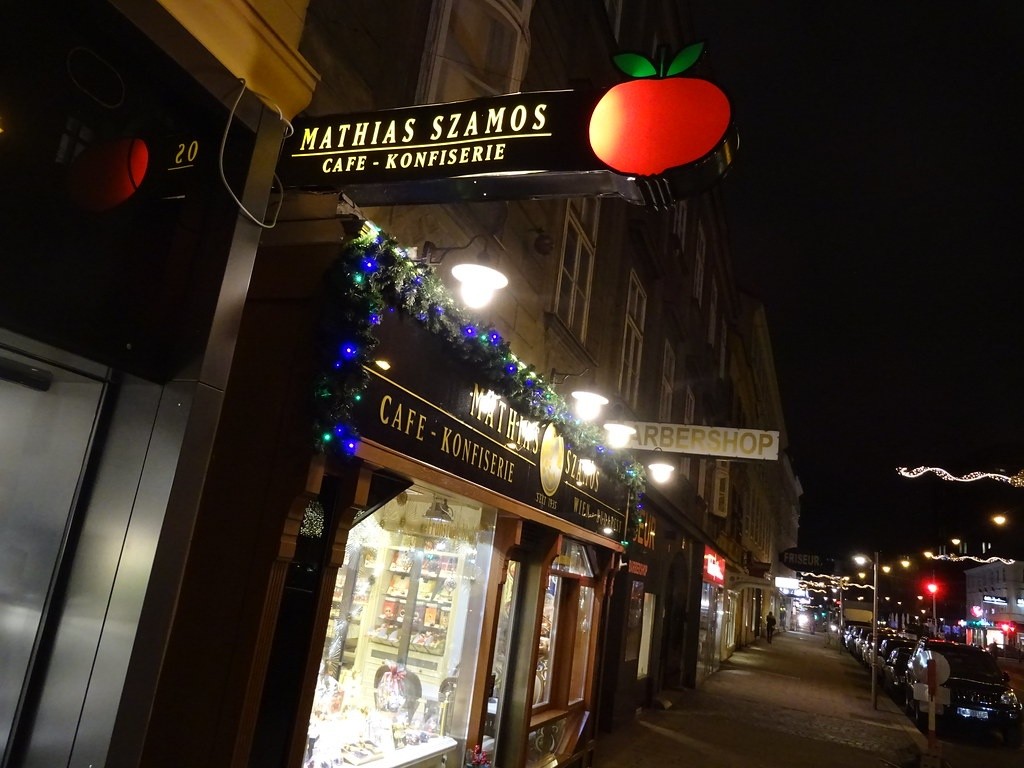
[423,233,508,309]
[550,367,674,483]
[424,497,452,522]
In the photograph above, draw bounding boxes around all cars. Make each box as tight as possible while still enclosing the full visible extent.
[856,628,874,656]
[881,647,914,704]
[844,625,867,651]
[876,639,918,677]
[981,643,1023,659]
[862,633,886,665]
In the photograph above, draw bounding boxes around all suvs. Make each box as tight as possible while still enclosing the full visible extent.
[904,636,1024,749]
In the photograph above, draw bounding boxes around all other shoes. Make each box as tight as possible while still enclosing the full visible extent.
[767,637,773,644]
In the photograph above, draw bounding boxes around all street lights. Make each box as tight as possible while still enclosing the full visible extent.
[852,551,879,708]
[928,569,936,618]
[939,616,945,630]
[831,583,842,654]
[823,595,834,620]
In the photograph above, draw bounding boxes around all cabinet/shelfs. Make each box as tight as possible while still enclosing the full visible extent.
[366,547,465,683]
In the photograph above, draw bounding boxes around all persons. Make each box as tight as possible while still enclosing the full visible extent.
[990,642,997,658]
[766,611,777,644]
